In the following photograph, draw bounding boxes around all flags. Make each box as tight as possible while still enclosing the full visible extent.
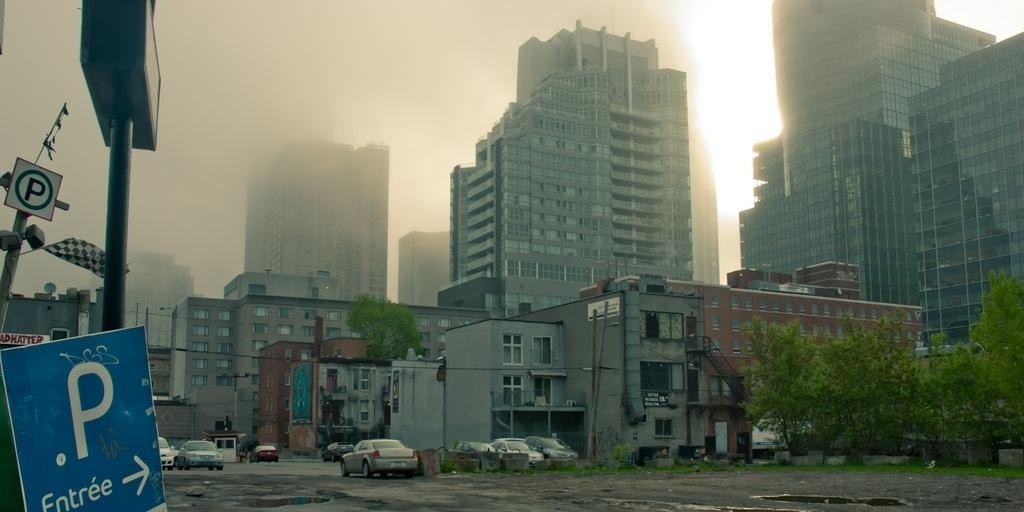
[42,237,129,280]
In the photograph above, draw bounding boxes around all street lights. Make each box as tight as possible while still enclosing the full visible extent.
[587,300,620,460]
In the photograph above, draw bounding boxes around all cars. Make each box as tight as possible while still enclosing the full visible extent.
[158,438,223,470]
[457,437,580,468]
[322,438,419,479]
[249,445,278,463]
